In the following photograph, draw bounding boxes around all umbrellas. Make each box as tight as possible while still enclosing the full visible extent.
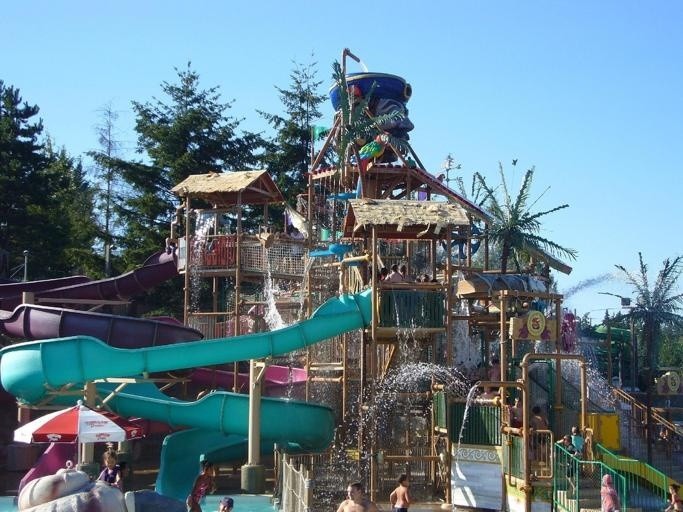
[13,399,144,471]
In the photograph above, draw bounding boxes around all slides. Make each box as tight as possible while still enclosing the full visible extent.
[1,249,255,503]
[1,248,373,502]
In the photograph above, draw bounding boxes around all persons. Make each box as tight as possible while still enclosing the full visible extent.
[377,263,437,283]
[186,459,217,511]
[478,353,585,498]
[336,482,380,512]
[664,484,683,512]
[600,474,621,512]
[218,497,233,512]
[117,462,129,491]
[98,450,124,491]
[390,474,416,512]
